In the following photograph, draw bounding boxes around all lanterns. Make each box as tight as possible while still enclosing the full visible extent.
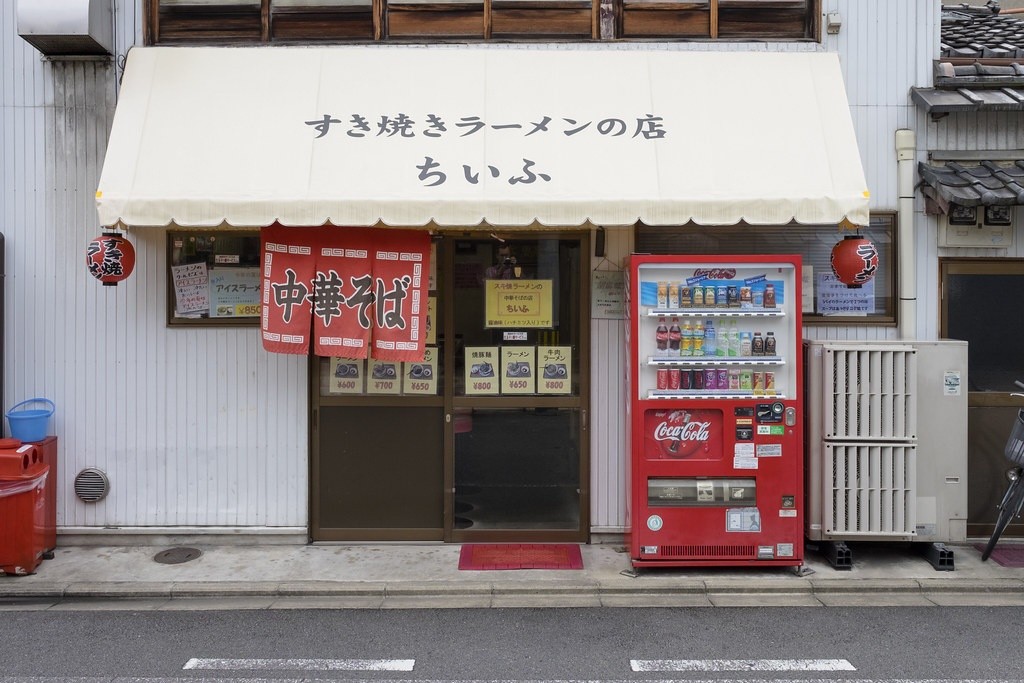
[86,233,136,286]
[830,235,879,288]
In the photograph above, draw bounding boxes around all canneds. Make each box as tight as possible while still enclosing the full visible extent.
[680,285,738,308]
[656,368,730,389]
[753,371,774,390]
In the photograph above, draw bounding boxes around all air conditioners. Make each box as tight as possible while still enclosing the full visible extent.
[804,340,969,542]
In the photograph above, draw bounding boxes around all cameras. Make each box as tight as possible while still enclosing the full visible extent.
[503,258,511,266]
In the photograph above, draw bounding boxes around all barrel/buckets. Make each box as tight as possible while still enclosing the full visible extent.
[4,397,56,443]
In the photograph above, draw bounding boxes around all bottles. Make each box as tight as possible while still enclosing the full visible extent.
[764,283,777,308]
[655,317,776,356]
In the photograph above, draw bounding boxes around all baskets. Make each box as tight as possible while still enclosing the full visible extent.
[1004,408,1024,467]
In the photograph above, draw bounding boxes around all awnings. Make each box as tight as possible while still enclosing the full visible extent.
[94,43,870,226]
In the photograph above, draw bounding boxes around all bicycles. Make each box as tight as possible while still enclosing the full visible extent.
[980,380,1024,563]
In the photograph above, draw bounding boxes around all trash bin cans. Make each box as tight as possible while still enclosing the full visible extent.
[0,438,51,574]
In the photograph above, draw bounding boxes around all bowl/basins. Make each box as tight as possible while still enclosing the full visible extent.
[470,363,566,376]
[387,368,394,376]
[424,368,431,377]
[336,364,349,375]
[350,368,357,374]
[412,364,423,377]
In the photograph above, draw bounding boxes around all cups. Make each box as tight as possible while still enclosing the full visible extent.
[657,368,728,389]
[656,280,763,307]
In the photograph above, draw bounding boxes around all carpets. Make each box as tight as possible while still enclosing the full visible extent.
[458,543,583,571]
